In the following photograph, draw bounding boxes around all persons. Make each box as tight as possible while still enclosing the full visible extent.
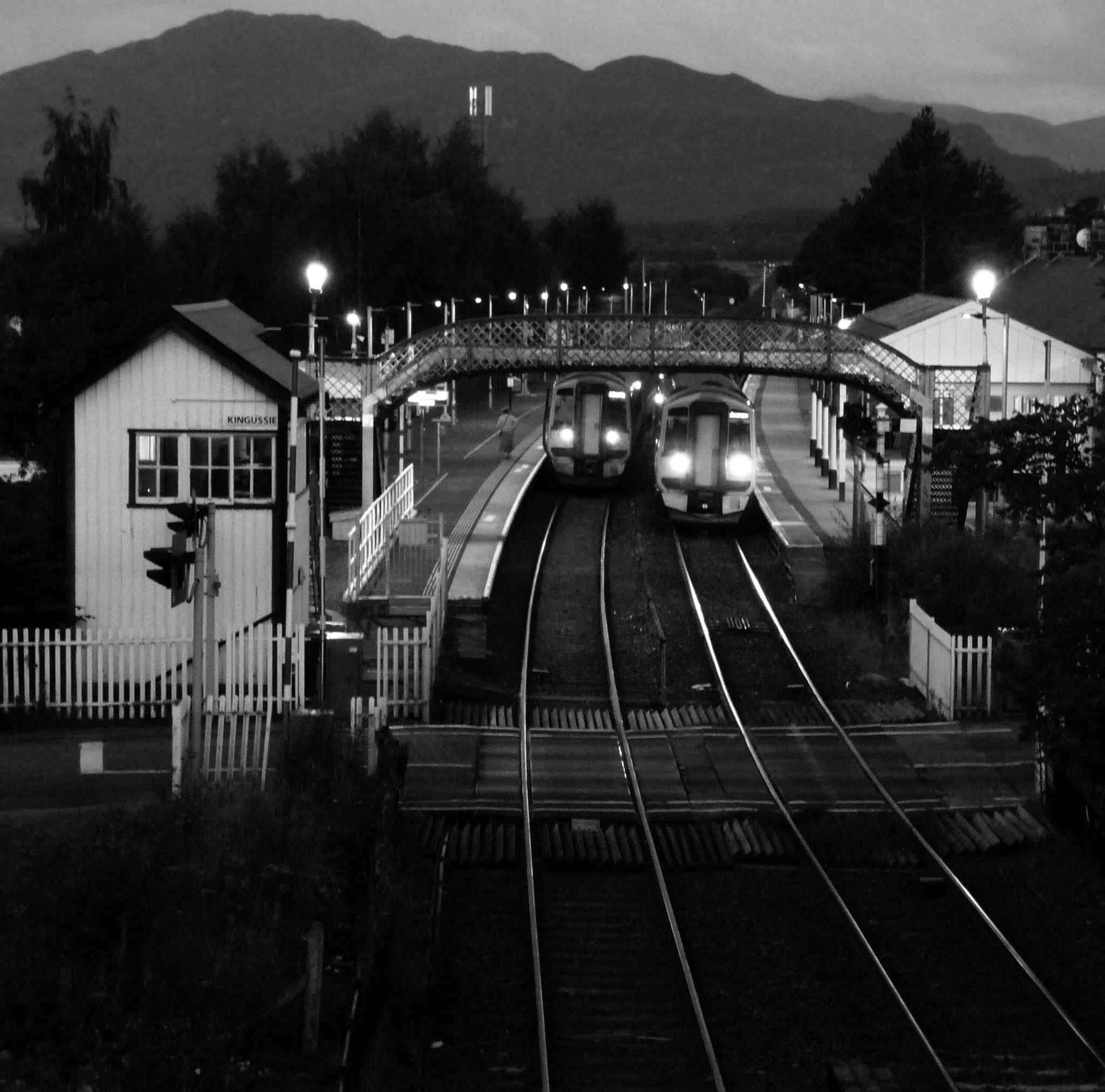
[496,407,520,461]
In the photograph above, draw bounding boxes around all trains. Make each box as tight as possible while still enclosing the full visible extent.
[639,371,758,529]
[542,370,655,483]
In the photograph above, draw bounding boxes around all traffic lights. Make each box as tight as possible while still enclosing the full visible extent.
[143,532,197,609]
[165,502,204,540]
